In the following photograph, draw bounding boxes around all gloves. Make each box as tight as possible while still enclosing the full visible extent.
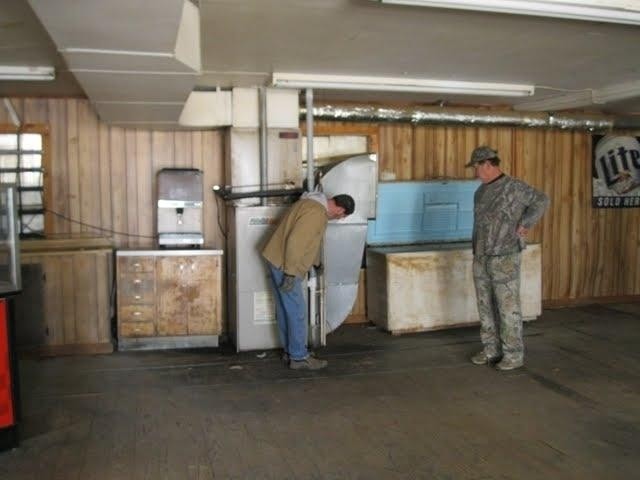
[276,273,296,292]
[312,262,325,276]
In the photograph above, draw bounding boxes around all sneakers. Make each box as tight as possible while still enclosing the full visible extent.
[494,357,525,370]
[470,351,503,366]
[281,351,328,370]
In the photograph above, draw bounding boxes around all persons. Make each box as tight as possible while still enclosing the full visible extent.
[464,146,548,372]
[262,191,353,369]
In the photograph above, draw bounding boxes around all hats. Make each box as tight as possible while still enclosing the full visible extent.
[464,146,498,168]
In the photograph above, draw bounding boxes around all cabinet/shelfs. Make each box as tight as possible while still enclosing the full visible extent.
[117,255,223,353]
